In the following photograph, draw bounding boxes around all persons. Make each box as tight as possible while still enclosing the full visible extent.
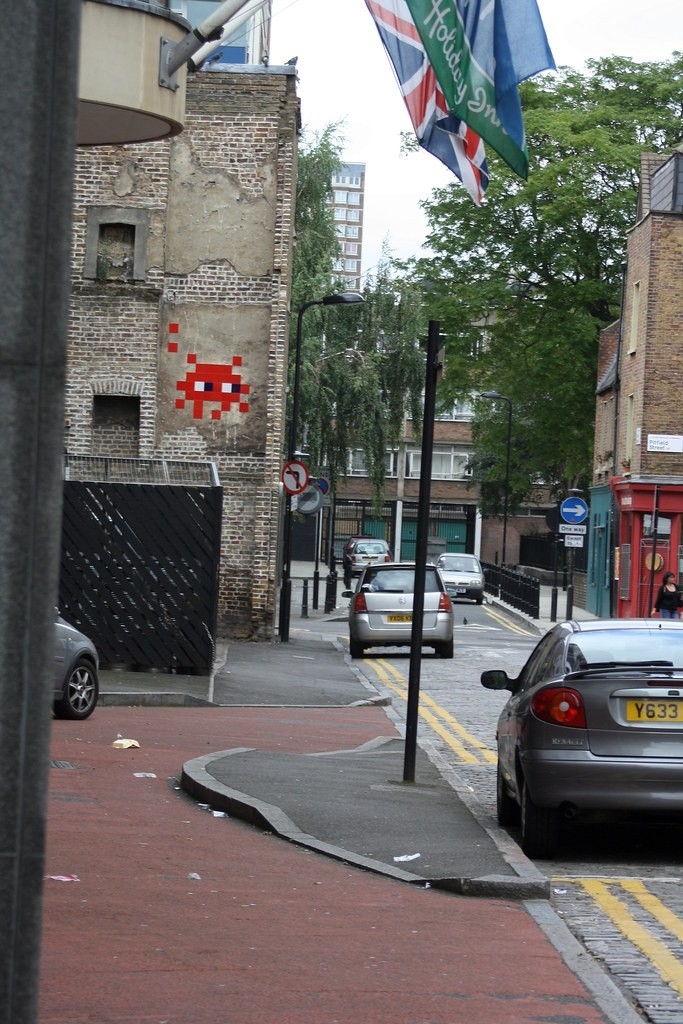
[650,571,681,619]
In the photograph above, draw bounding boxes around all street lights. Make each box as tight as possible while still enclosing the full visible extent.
[480,391,512,600]
[280,293,366,641]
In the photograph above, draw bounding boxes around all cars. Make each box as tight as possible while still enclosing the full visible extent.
[436,553,486,605]
[480,617,683,861]
[343,536,393,577]
[342,562,454,659]
[52,608,100,720]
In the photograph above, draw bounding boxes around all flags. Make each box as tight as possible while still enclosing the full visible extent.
[405,0,558,183]
[365,0,490,209]
